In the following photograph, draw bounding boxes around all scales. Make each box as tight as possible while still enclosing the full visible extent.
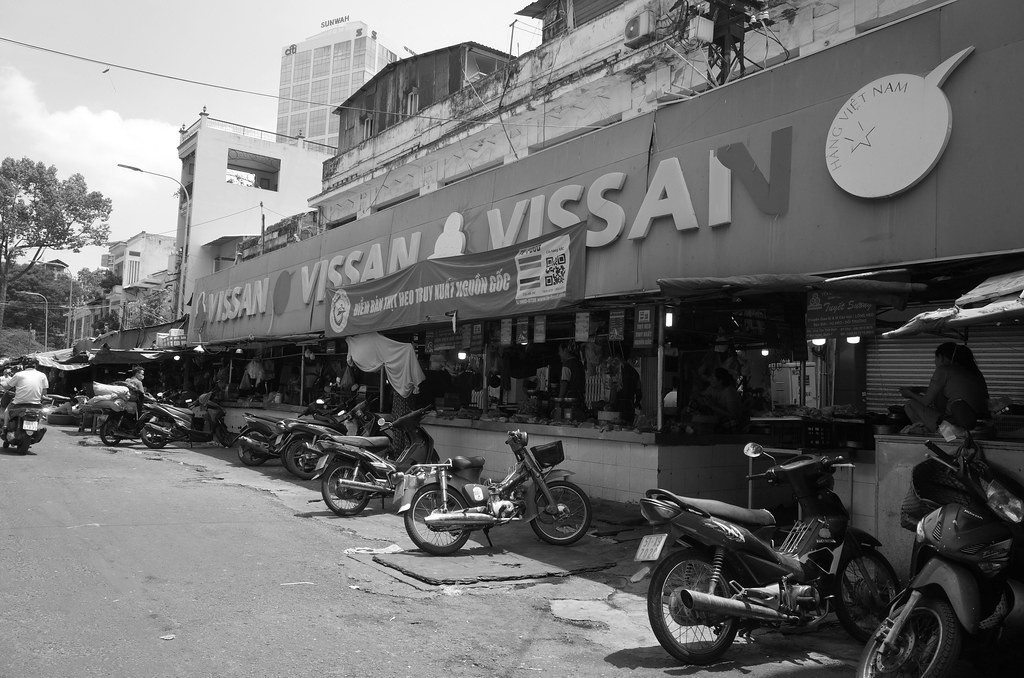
[554,397,580,421]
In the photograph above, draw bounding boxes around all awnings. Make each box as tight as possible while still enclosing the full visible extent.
[53,315,189,366]
[189,330,323,351]
[24,346,92,372]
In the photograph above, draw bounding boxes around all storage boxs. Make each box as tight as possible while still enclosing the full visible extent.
[48,414,74,425]
[598,411,623,420]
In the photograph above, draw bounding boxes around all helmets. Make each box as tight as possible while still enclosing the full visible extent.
[21,353,39,369]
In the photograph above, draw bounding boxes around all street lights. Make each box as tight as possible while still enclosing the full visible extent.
[29,260,73,349]
[14,291,48,352]
[118,163,190,321]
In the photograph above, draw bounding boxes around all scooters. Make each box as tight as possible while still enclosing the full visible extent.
[851,406,1024,678]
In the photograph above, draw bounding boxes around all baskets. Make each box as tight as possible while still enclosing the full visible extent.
[530,440,565,467]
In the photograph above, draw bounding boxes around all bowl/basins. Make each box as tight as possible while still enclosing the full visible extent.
[899,386,921,394]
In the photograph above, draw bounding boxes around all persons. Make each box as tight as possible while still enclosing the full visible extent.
[0,360,49,429]
[0,367,12,382]
[664,361,741,420]
[52,397,81,419]
[287,367,300,404]
[898,344,989,434]
[125,366,145,394]
[392,387,421,461]
[558,340,587,405]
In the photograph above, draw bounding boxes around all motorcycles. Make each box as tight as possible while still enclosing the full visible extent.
[68,375,389,479]
[631,442,901,666]
[311,403,441,517]
[390,427,593,556]
[0,384,48,454]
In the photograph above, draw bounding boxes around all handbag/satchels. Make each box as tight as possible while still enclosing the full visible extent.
[901,452,1024,534]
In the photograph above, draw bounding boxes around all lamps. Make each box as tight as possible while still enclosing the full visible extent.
[458,349,466,359]
[235,344,243,353]
[193,343,204,352]
[305,346,315,360]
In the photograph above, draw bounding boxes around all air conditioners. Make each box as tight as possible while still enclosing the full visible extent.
[624,9,656,46]
